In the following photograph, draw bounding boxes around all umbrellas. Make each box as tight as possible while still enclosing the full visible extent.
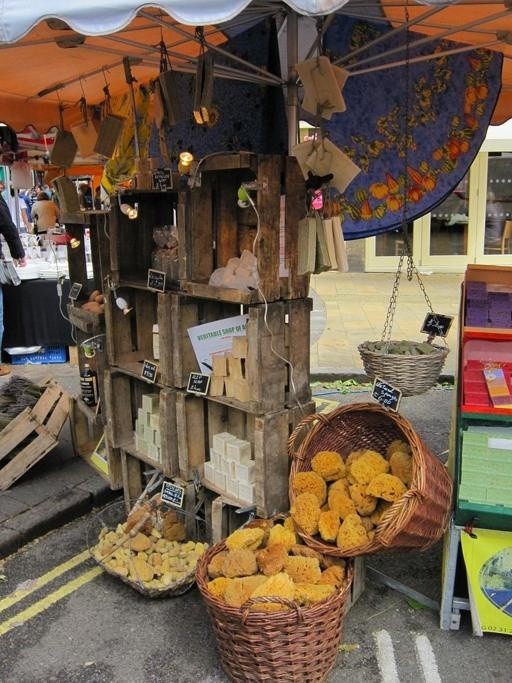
[0,0,512,164]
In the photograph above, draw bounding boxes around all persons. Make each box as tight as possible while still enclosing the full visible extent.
[483,187,506,255]
[0,180,101,238]
[448,194,470,254]
[0,193,27,377]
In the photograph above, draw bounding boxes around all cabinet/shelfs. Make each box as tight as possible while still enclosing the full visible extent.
[68,152,315,545]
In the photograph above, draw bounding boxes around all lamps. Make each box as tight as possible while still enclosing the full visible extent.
[237,183,251,209]
[120,203,139,220]
[68,233,80,249]
[177,150,194,177]
[108,280,133,316]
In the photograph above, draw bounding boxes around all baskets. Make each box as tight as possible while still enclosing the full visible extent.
[360,341,449,397]
[87,499,211,595]
[287,402,453,558]
[12,344,67,363]
[197,514,354,683]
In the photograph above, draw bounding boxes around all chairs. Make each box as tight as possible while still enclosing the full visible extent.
[394,240,404,256]
[485,220,512,254]
